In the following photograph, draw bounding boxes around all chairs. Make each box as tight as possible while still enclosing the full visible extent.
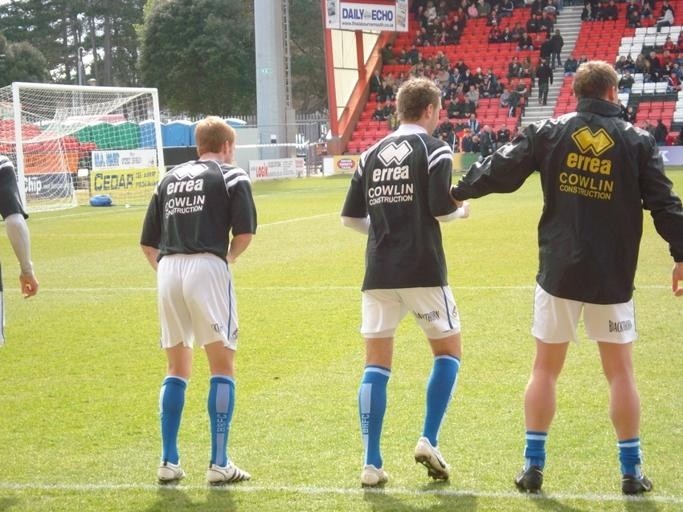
[346,0,681,149]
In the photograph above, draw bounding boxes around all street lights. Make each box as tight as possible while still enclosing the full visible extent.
[75,46,86,108]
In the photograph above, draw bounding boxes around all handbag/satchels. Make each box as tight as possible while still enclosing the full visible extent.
[88,193,112,207]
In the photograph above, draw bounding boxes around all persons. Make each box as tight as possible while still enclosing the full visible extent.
[0,155,38,350]
[140,116,256,484]
[449,60,683,493]
[340,77,471,487]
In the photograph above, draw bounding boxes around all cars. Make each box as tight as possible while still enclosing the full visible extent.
[147,110,204,128]
[296,123,331,157]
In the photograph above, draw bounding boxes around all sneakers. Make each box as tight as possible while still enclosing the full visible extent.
[621,470,654,497]
[358,461,389,487]
[413,435,450,480]
[205,456,252,487]
[156,456,186,483]
[513,464,545,495]
